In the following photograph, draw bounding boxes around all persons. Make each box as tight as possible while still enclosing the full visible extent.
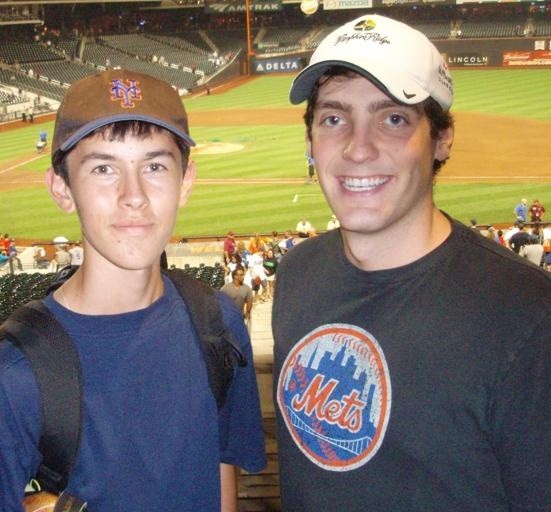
[219,214,341,327]
[34,130,48,153]
[0,68,270,512]
[304,153,319,184]
[0,1,551,121]
[272,14,550,512]
[470,197,551,271]
[0,231,84,273]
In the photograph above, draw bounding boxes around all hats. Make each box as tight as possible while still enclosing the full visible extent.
[288,12,454,116]
[52,69,195,164]
